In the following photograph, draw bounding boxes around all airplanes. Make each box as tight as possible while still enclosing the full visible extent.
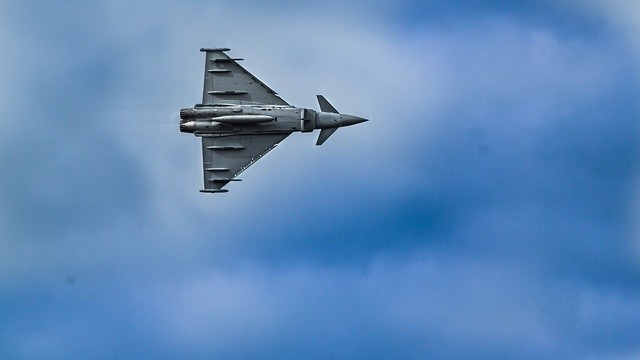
[179,46,369,193]
